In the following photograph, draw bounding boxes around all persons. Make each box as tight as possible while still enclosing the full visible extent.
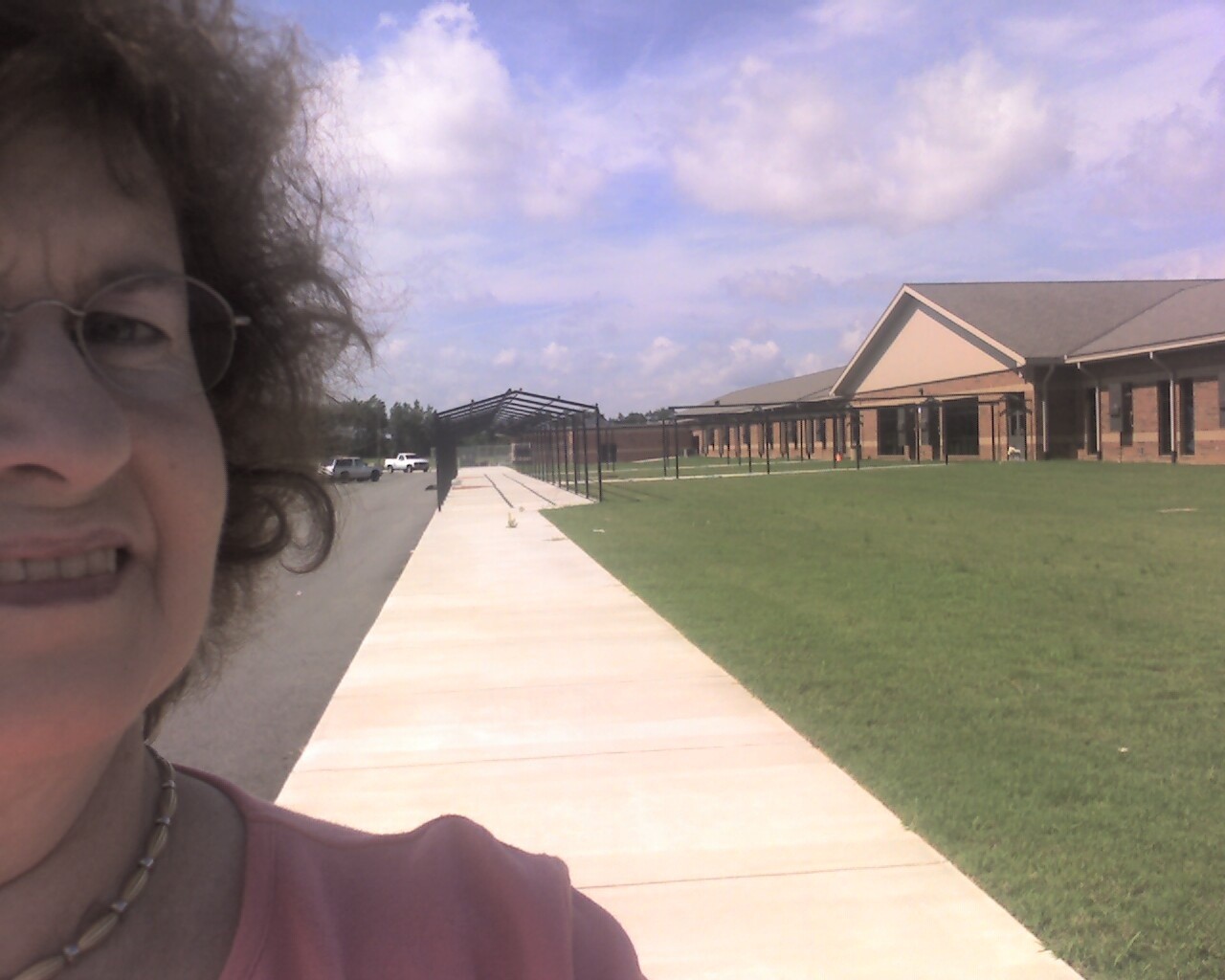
[0,0,640,980]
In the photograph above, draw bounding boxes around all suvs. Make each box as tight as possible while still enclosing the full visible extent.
[317,457,384,485]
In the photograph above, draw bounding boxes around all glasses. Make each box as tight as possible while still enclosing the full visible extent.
[0,272,251,402]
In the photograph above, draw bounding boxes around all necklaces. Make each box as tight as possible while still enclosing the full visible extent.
[11,742,178,980]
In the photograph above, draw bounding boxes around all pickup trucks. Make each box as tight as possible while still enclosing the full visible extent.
[383,452,430,474]
